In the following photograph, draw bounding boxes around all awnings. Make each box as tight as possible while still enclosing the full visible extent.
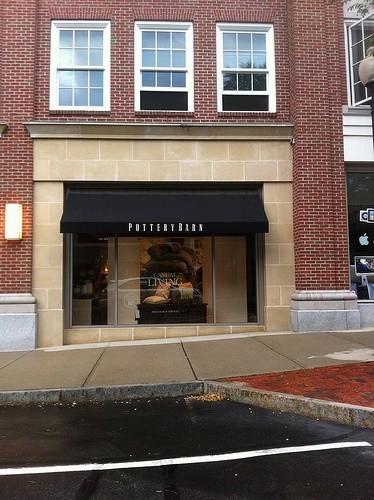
[57,185,270,234]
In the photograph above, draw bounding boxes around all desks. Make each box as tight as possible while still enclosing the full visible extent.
[136,302,209,325]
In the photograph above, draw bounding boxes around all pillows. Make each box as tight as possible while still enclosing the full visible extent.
[154,281,171,300]
[143,295,172,305]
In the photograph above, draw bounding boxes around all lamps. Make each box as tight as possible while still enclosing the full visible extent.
[4,203,23,241]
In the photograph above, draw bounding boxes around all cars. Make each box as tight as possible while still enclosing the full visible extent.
[101,276,203,304]
